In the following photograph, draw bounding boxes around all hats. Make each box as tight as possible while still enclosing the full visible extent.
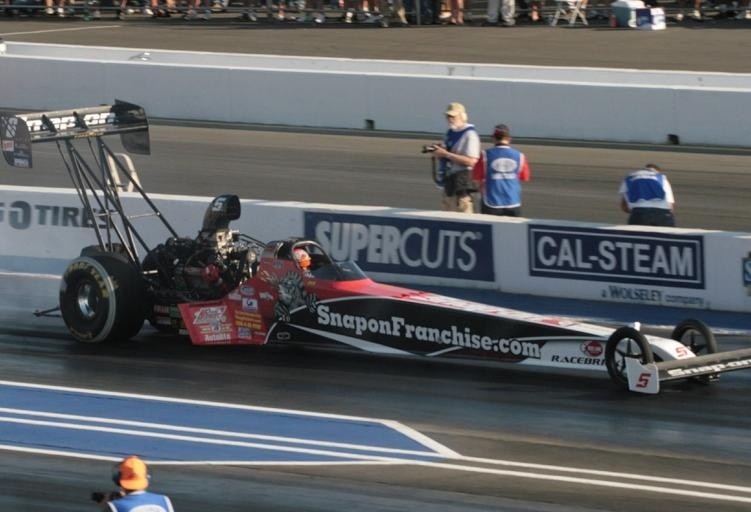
[118,455,148,490]
[491,124,510,137]
[444,102,465,116]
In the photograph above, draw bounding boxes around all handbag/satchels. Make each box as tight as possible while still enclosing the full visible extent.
[442,170,480,196]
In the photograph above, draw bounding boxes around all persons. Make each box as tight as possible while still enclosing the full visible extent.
[618,163,676,227]
[431,102,480,213]
[470,125,530,216]
[103,456,174,512]
[294,248,312,270]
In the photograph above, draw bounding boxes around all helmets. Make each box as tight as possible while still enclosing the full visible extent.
[293,249,311,267]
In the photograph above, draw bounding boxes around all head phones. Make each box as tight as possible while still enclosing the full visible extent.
[457,104,468,122]
[111,462,149,487]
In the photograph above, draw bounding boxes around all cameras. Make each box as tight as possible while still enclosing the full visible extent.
[423,144,447,153]
[92,491,122,503]
